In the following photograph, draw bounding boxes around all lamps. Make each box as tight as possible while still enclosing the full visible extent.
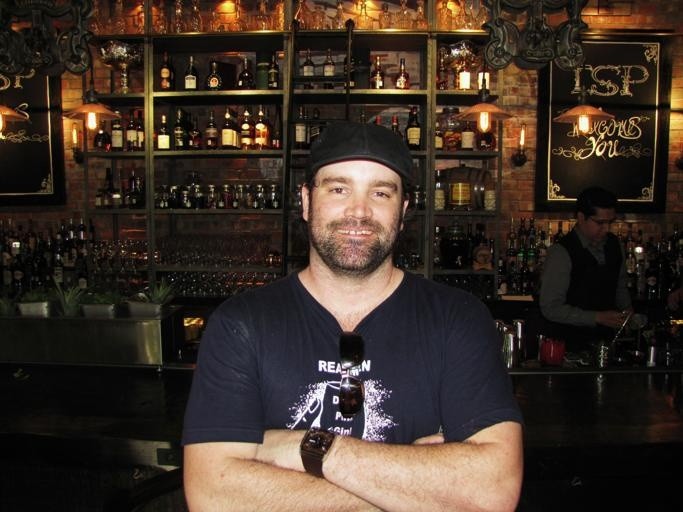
[554,60,615,133]
[73,124,84,164]
[512,125,526,166]
[0,105,26,131]
[61,35,122,130]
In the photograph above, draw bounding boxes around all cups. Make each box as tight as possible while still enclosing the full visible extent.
[646,346,657,366]
[276,4,284,31]
[597,346,608,368]
[514,319,525,362]
[539,339,563,364]
[493,321,514,370]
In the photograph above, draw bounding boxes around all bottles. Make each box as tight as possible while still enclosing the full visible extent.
[268,50,279,89]
[237,58,253,89]
[89,239,282,296]
[172,110,184,149]
[436,0,452,32]
[0,217,88,295]
[153,0,168,35]
[303,49,315,90]
[254,0,272,31]
[353,0,372,30]
[93,121,110,148]
[256,62,268,90]
[126,111,144,151]
[498,216,683,296]
[206,62,221,90]
[371,57,384,89]
[434,106,496,152]
[331,3,346,29]
[119,64,127,93]
[137,2,144,34]
[228,0,246,32]
[107,1,126,35]
[293,105,422,150]
[294,2,309,29]
[207,6,221,33]
[221,103,280,150]
[395,59,409,90]
[344,49,356,88]
[431,275,492,303]
[156,113,170,149]
[412,1,427,31]
[205,112,218,150]
[93,164,145,209]
[378,4,391,30]
[413,185,422,209]
[183,57,198,90]
[158,51,171,89]
[433,218,494,270]
[323,49,335,91]
[111,111,123,151]
[293,183,301,206]
[188,116,200,149]
[83,1,104,36]
[189,2,201,33]
[438,49,489,89]
[396,229,419,268]
[157,184,280,210]
[311,6,325,30]
[454,1,491,30]
[170,1,187,35]
[434,170,496,212]
[395,0,411,29]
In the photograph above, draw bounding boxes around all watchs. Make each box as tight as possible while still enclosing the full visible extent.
[297,426,338,482]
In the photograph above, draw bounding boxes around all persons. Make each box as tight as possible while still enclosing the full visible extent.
[183,120,525,512]
[534,187,630,352]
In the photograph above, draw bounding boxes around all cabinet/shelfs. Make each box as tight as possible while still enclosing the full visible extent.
[80,0,504,319]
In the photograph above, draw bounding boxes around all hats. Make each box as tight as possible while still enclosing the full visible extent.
[305,121,413,183]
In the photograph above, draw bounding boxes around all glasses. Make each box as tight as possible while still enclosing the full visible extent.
[339,332,365,418]
